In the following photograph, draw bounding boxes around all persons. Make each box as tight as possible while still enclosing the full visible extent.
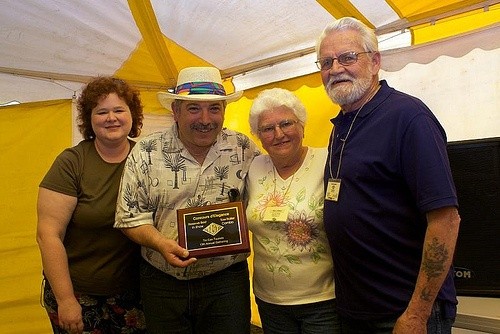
[113,67,263,334]
[314,17,461,334]
[36,76,145,334]
[245,88,337,333]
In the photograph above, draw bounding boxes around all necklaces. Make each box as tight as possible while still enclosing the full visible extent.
[189,149,208,156]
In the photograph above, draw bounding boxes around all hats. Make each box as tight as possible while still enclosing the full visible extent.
[157,67,244,112]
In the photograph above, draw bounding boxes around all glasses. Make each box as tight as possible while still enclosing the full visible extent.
[315,51,372,70]
